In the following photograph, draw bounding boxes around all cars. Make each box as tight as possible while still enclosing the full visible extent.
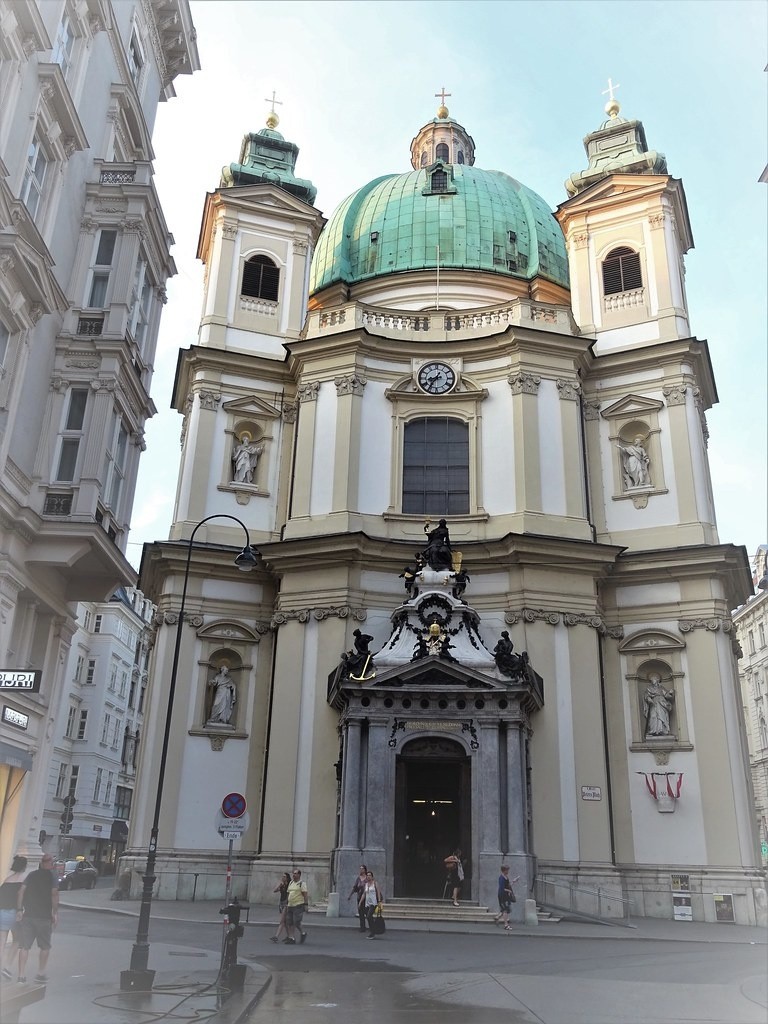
[57,856,99,890]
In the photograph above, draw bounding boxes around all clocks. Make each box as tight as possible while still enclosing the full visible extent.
[417,363,455,395]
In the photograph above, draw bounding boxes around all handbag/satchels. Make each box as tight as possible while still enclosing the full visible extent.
[446,856,456,870]
[507,885,516,903]
[372,906,386,934]
[374,881,383,902]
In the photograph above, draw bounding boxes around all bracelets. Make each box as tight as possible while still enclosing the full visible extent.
[16,908,24,912]
[304,904,308,906]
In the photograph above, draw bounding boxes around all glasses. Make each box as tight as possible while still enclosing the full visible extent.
[283,875,286,878]
[293,873,300,875]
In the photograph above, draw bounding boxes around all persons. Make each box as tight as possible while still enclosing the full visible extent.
[335,628,375,677]
[409,635,433,663]
[615,439,651,488]
[493,629,528,676]
[643,673,676,735]
[0,853,59,985]
[438,636,460,664]
[269,870,309,945]
[207,666,238,724]
[493,864,520,931]
[232,436,265,484]
[347,865,382,939]
[399,520,470,595]
[444,850,465,906]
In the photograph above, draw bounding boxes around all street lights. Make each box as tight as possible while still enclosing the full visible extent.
[118,514,258,992]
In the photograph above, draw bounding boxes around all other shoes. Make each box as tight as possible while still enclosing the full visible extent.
[2,968,13,979]
[453,902,460,906]
[270,936,278,943]
[34,974,49,982]
[300,931,307,944]
[285,939,296,944]
[359,927,366,932]
[366,934,376,940]
[504,924,512,931]
[18,976,27,985]
[282,937,289,942]
[451,896,458,901]
[494,916,499,925]
